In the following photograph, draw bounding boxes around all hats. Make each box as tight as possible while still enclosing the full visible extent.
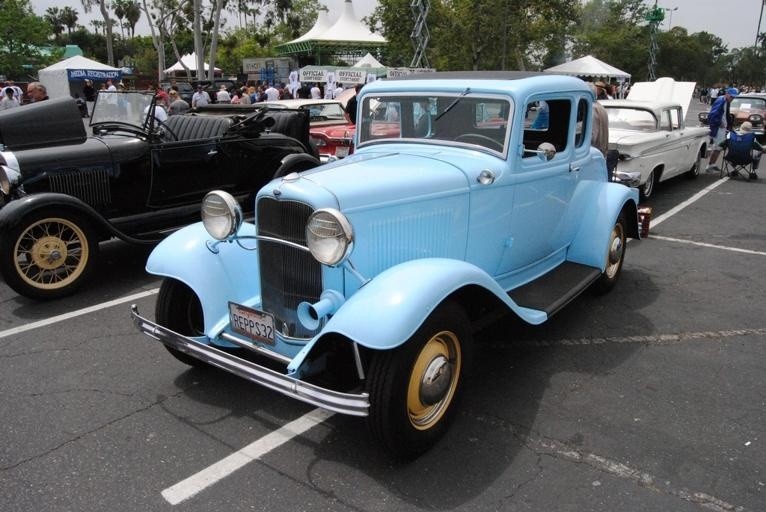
[740,121,753,134]
[727,88,737,98]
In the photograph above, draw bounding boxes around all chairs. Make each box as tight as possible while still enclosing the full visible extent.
[719,130,756,179]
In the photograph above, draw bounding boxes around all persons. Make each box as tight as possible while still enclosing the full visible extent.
[345,84,433,130]
[1,79,49,111]
[142,79,345,130]
[718,122,766,182]
[74,78,123,107]
[523,74,766,173]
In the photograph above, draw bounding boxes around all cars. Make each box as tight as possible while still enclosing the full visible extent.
[726,93,766,140]
[322,86,388,122]
[591,77,712,202]
[249,98,399,162]
[159,76,235,105]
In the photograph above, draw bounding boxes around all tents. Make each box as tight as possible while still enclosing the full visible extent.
[35,55,123,107]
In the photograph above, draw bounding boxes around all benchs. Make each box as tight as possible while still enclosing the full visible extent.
[240,112,301,138]
[159,116,228,144]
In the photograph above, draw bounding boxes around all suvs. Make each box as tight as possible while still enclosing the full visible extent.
[0,88,320,301]
[130,69,652,458]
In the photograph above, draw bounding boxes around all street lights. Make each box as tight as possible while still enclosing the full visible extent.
[665,6,679,32]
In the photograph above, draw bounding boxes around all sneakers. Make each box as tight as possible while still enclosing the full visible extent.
[704,164,757,179]
